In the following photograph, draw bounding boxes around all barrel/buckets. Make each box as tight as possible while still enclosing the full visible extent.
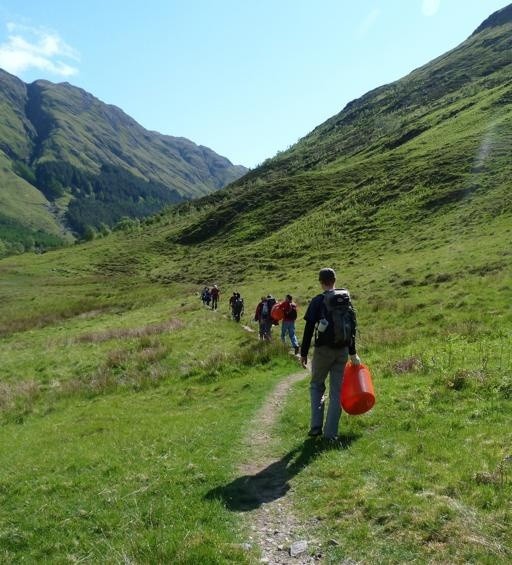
[270,302,285,320]
[340,360,377,415]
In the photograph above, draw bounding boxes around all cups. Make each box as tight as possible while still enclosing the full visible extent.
[318,319,328,332]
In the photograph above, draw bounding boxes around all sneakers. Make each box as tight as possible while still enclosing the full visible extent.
[307,427,323,437]
[294,345,300,355]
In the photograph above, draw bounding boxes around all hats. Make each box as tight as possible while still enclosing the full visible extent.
[318,266,336,283]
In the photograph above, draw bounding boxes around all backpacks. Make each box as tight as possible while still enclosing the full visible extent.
[283,303,298,320]
[315,290,358,350]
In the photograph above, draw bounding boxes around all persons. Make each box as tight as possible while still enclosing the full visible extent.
[234,292,245,323]
[300,268,360,440]
[271,294,300,355]
[255,296,274,341]
[205,289,212,305]
[265,293,276,307]
[228,292,236,316]
[201,287,208,304]
[210,284,220,310]
